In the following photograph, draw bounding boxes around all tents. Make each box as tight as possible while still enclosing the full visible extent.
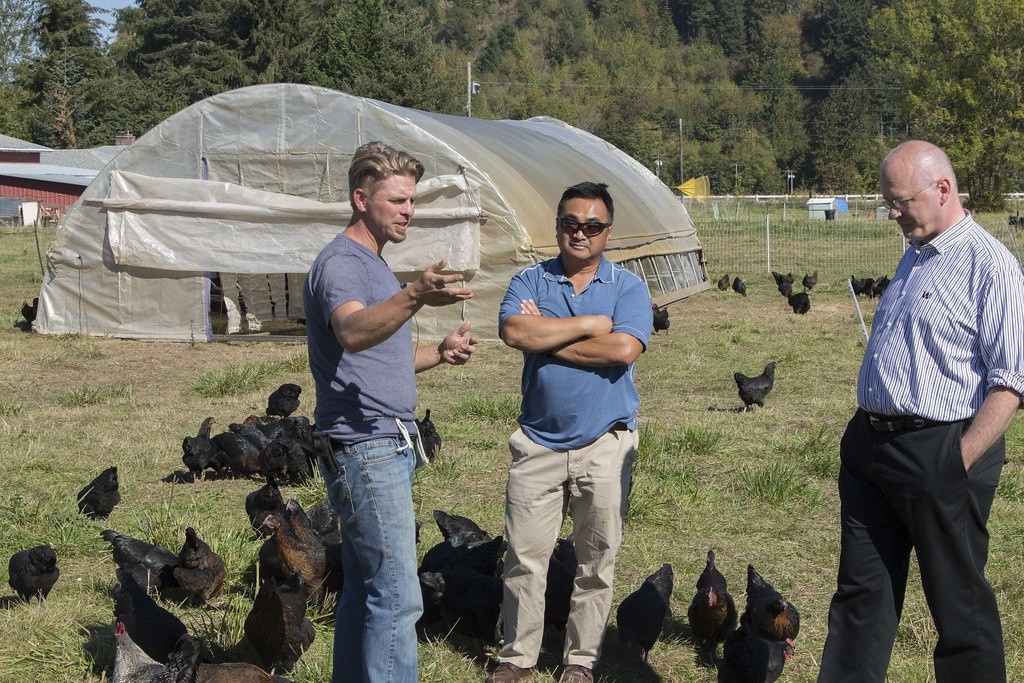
[35,81,711,339]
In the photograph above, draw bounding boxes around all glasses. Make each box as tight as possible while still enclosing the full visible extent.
[882,180,940,209]
[560,218,612,237]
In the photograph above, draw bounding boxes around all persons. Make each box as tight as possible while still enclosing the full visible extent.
[300,137,478,683]
[487,183,653,683]
[812,141,1024,683]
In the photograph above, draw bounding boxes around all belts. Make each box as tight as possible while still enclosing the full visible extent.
[328,438,344,450]
[867,415,970,432]
[612,422,626,430]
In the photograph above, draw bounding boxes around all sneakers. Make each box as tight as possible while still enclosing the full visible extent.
[485,662,535,683]
[562,665,593,683]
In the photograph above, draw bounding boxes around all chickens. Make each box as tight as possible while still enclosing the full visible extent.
[98,526,297,683]
[20,298,39,325]
[686,548,740,666]
[739,565,800,642]
[245,477,422,608]
[772,270,793,295]
[77,466,123,521]
[616,561,673,669]
[734,361,777,415]
[787,292,810,314]
[849,273,890,299]
[8,545,61,601]
[242,571,318,675]
[652,303,671,335]
[718,274,729,291]
[716,632,796,683]
[732,277,746,296]
[802,269,819,291]
[182,385,442,481]
[416,506,577,647]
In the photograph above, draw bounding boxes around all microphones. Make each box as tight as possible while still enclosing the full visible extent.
[400,283,407,289]
